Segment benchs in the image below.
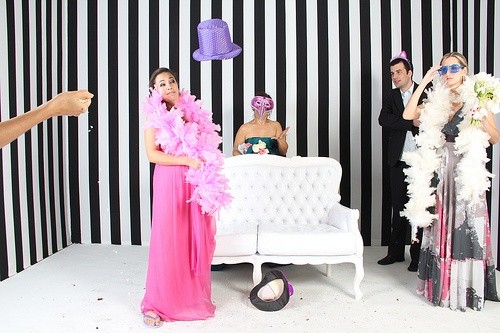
[211,153,364,301]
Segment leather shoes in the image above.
[377,256,406,265]
[407,260,419,271]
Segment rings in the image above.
[85,107,87,110]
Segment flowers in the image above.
[469,78,499,125]
[237,140,269,155]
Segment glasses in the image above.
[438,65,464,75]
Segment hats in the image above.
[249,269,293,312]
[193,19,242,61]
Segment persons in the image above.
[0,90,94,148]
[233,93,290,157]
[140,67,235,329]
[402,53,500,311]
[376,59,427,272]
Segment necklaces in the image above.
[142,86,232,215]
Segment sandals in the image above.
[142,313,160,327]
